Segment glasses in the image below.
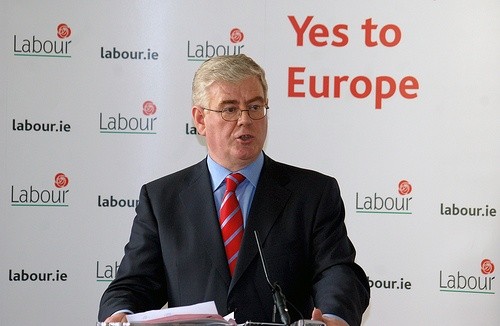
[204,104,269,122]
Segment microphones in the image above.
[254,230,275,290]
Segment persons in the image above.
[98,54,371,326]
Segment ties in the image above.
[219,174,247,276]
